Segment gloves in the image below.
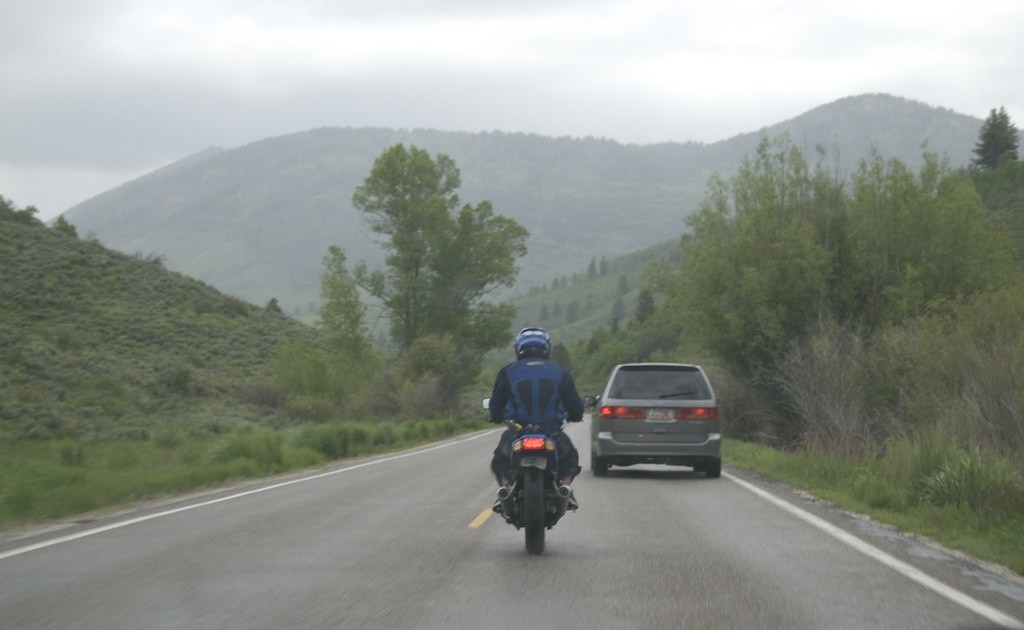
[566,410,581,423]
[489,412,506,424]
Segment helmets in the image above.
[515,326,551,359]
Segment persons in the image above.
[489,327,584,510]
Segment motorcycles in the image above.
[481,394,600,553]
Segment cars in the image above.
[591,363,722,480]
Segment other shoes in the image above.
[492,496,516,511]
[568,492,579,510]
